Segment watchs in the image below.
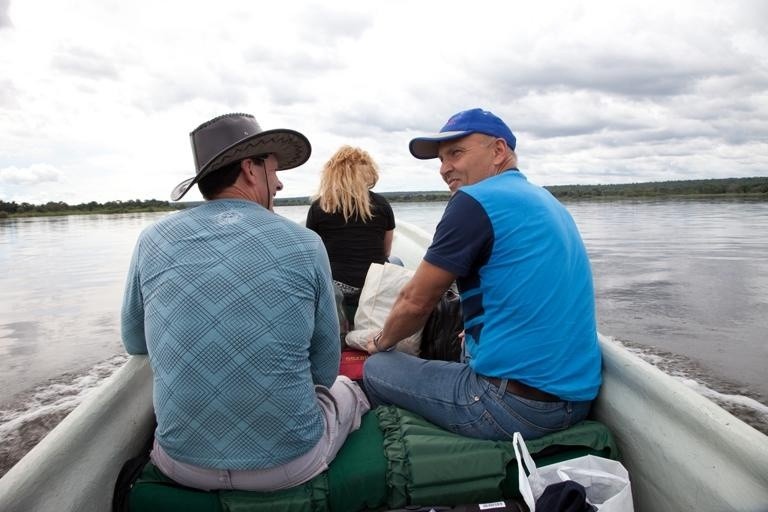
[373,328,396,352]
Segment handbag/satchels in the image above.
[343,262,463,361]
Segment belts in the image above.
[479,374,560,402]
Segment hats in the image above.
[170,113,311,201]
[409,108,516,159]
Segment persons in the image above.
[120,113,371,493]
[363,108,602,441]
[305,144,405,325]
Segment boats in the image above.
[2,217,768,511]
